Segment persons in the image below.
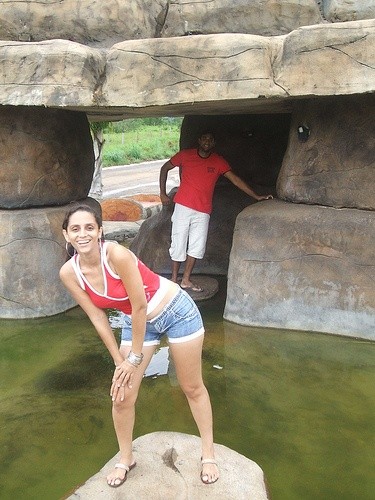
[59,204,219,488]
[159,130,273,292]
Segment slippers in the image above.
[201,457,219,484]
[181,280,204,292]
[176,282,180,285]
[107,459,136,488]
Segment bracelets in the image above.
[128,352,144,366]
